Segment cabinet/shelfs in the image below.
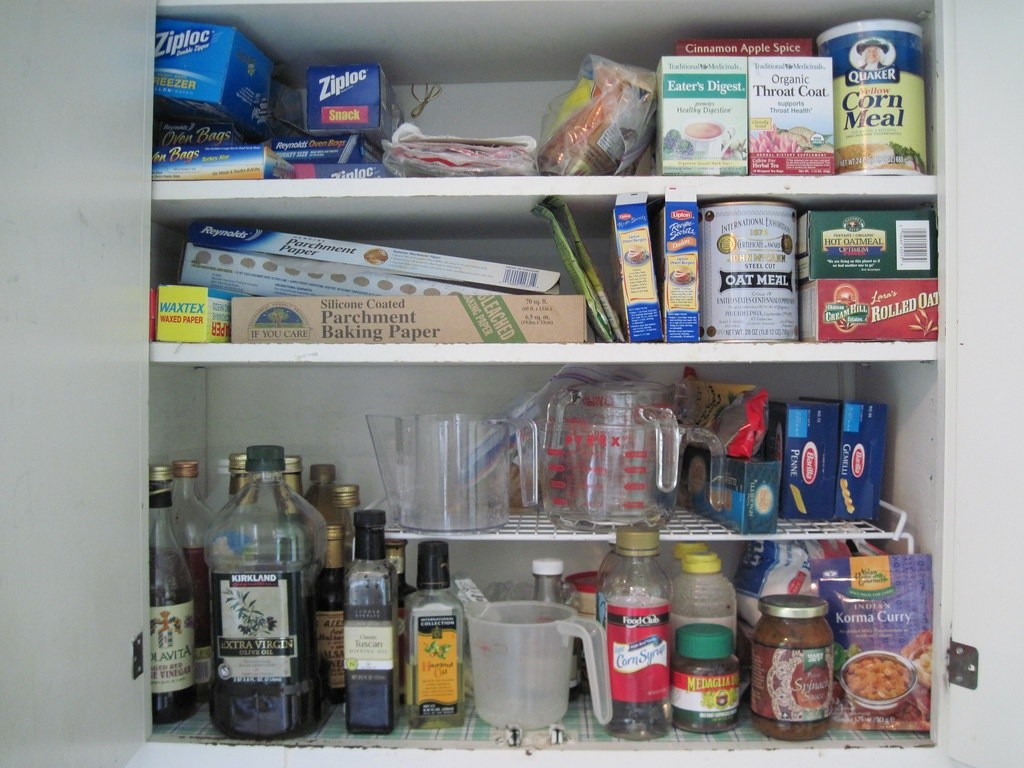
[147,0,942,759]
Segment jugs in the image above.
[545,380,726,527]
[366,412,539,531]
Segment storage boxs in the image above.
[649,16,929,178]
[151,16,405,181]
[677,396,890,536]
[795,208,938,282]
[650,189,702,343]
[615,189,661,341]
[694,199,802,340]
[152,221,598,344]
[798,275,939,342]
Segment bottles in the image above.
[148,444,830,742]
